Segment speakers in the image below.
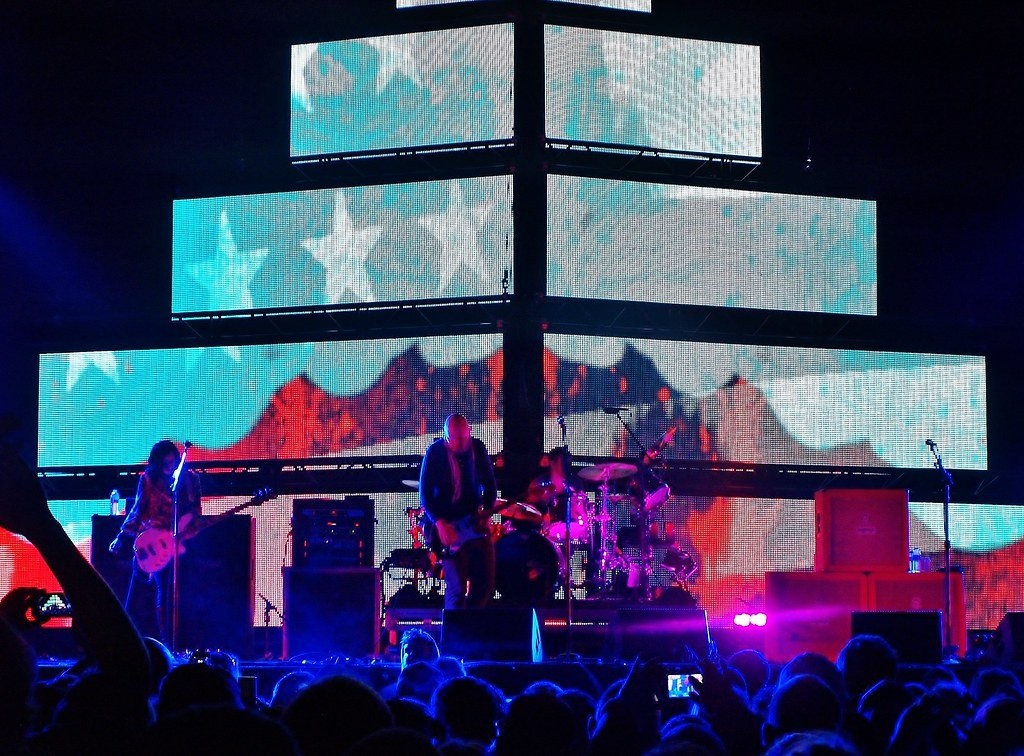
[986,612,1024,667]
[812,489,909,573]
[282,567,383,661]
[851,611,943,665]
[89,515,254,656]
[867,573,966,659]
[764,570,868,662]
[438,608,543,663]
[604,609,711,660]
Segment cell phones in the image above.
[238,677,258,709]
[32,593,73,618]
[663,673,703,699]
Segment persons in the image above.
[0,448,152,756]
[108,440,201,645]
[540,447,590,576]
[419,413,496,609]
[127,589,1024,756]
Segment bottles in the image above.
[909,546,921,572]
[109,489,120,515]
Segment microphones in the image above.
[602,408,628,413]
[926,439,937,445]
[557,415,564,423]
